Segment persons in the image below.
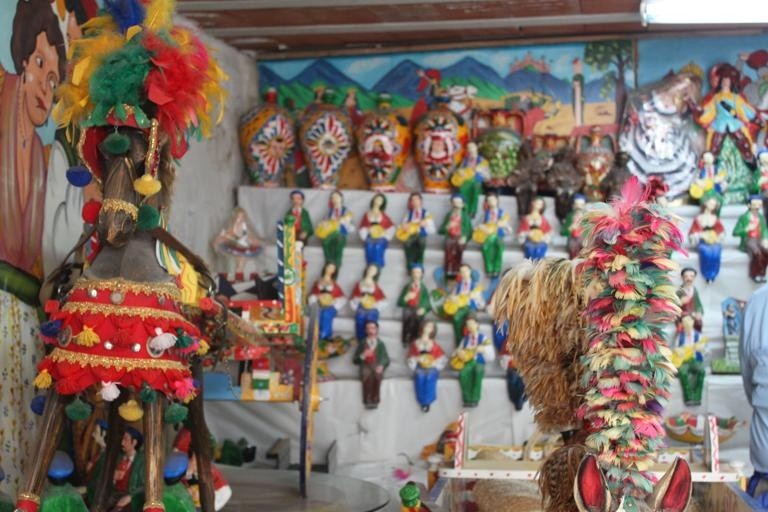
[689,151,727,217]
[675,268,704,333]
[284,191,313,256]
[450,142,490,219]
[443,264,484,348]
[315,189,355,279]
[0,1,68,283]
[397,192,436,273]
[438,193,473,273]
[161,453,201,511]
[397,264,431,348]
[683,61,766,172]
[516,194,553,262]
[353,320,389,410]
[87,421,116,510]
[211,207,266,282]
[472,189,515,279]
[560,193,591,261]
[670,313,708,406]
[688,191,727,285]
[349,264,387,341]
[108,426,147,511]
[359,193,396,268]
[733,195,767,285]
[449,312,496,407]
[406,319,448,414]
[499,332,529,412]
[306,262,349,340]
[738,284,768,472]
[38,450,87,511]
[749,149,768,194]
